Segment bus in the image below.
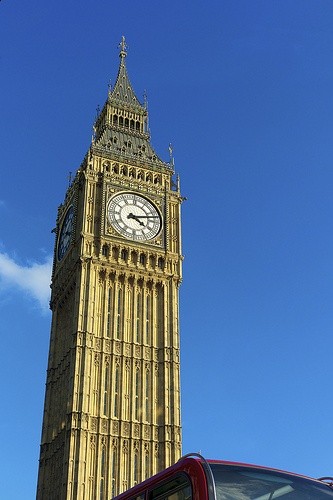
[104,453,331,500]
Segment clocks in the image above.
[107,190,165,242]
[56,204,80,259]
